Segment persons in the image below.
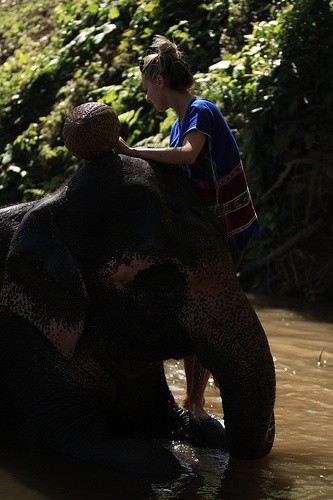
[109,34,264,424]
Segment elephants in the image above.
[0,146,277,484]
